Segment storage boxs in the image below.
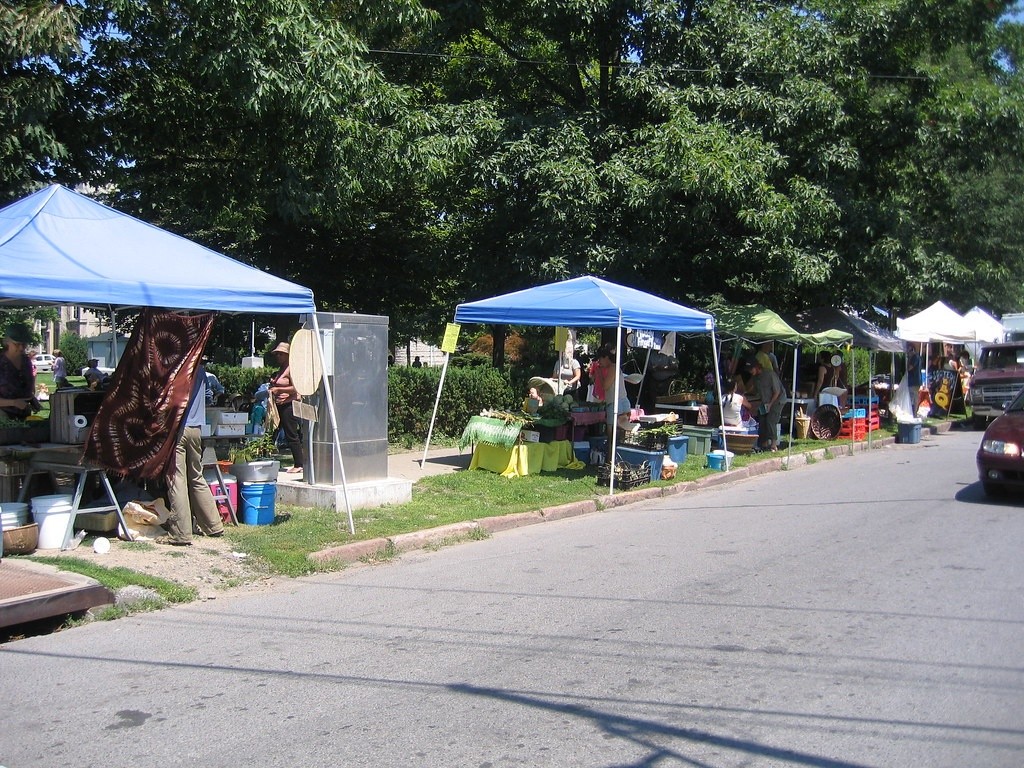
[664,436,689,466]
[835,394,880,441]
[202,468,237,523]
[615,446,667,482]
[201,425,211,436]
[206,411,249,425]
[73,510,119,531]
[211,425,245,436]
[682,425,714,455]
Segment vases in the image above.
[719,433,759,456]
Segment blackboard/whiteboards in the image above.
[930,369,968,417]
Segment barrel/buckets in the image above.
[31,494,75,549]
[240,482,277,525]
[706,450,733,470]
[0,503,39,558]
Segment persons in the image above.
[755,338,779,373]
[48,350,74,389]
[552,355,581,393]
[250,340,304,473]
[814,350,843,408]
[722,380,751,433]
[26,350,38,396]
[904,342,921,417]
[0,323,59,493]
[412,356,422,368]
[84,359,109,389]
[388,355,393,367]
[156,361,226,543]
[522,388,544,406]
[588,342,631,462]
[745,356,787,452]
[917,343,973,419]
[36,382,50,400]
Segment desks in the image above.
[0,428,265,455]
[785,398,817,437]
[654,403,720,426]
[459,410,608,477]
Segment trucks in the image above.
[1000,312,1024,363]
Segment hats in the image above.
[272,342,290,353]
[593,346,610,362]
[7,324,34,343]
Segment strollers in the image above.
[527,376,569,402]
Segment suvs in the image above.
[967,340,1024,430]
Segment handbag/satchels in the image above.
[265,389,280,431]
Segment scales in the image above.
[621,333,645,385]
[821,355,847,398]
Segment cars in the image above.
[33,353,56,366]
[975,386,1024,496]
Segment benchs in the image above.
[15,451,134,543]
[198,440,240,528]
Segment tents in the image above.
[888,301,998,423]
[0,182,355,535]
[964,306,1010,359]
[419,275,729,495]
[798,302,910,452]
[688,290,857,468]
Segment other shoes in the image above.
[288,467,304,473]
[156,534,191,546]
[193,525,224,537]
[769,445,778,453]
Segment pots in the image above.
[229,461,280,481]
